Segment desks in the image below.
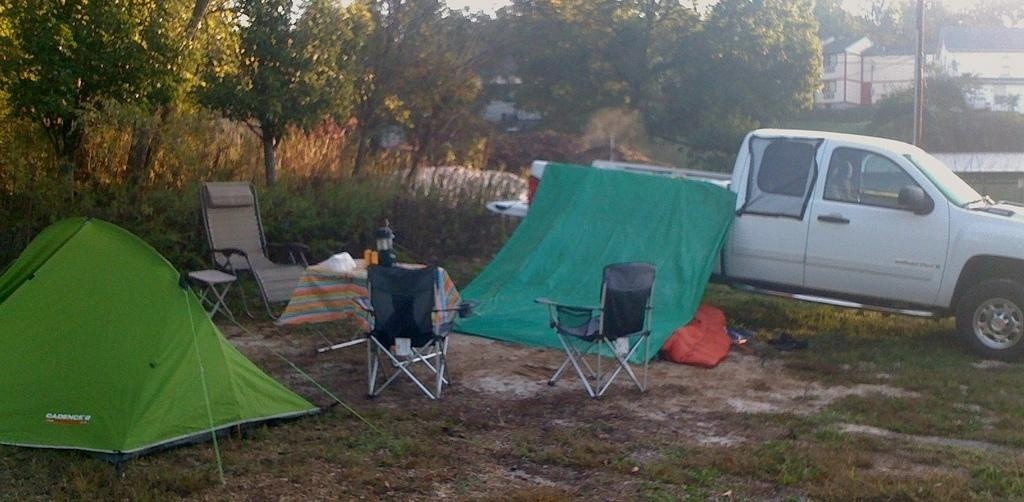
[273,257,465,369]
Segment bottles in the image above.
[363,249,378,270]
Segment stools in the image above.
[186,268,239,325]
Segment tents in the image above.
[1,218,324,458]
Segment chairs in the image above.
[533,260,656,400]
[197,177,315,323]
[351,260,482,404]
[822,161,858,204]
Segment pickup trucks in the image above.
[485,124,1024,364]
